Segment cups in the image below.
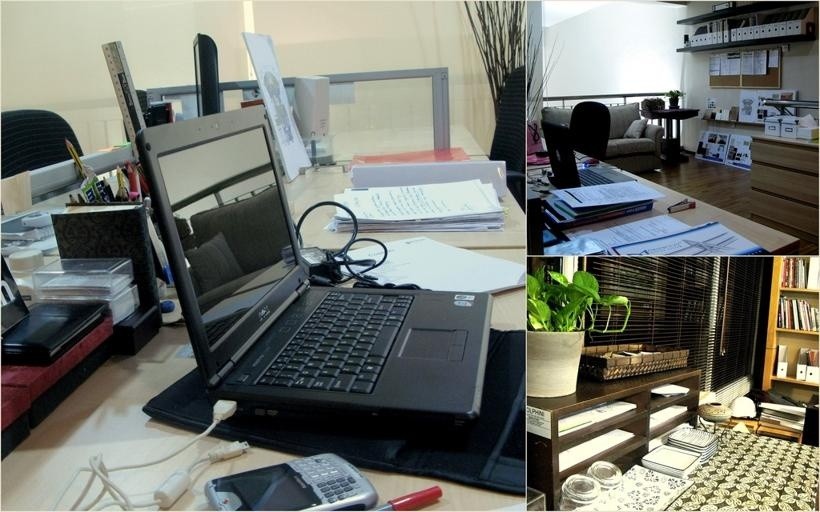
[587,463,624,511]
[561,474,602,512]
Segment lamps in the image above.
[293,74,338,168]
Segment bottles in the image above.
[10,249,47,310]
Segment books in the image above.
[541,183,652,230]
[707,16,754,33]
[778,258,819,366]
[760,401,805,432]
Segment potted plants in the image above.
[664,88,686,109]
[527,259,635,400]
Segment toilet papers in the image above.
[796,114,819,140]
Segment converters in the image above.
[299,246,332,277]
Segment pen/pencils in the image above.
[62,134,148,204]
[376,485,442,511]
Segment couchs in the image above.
[539,99,666,177]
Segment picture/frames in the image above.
[752,88,799,126]
[241,28,312,185]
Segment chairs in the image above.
[489,66,525,214]
[566,101,612,162]
[1,108,87,209]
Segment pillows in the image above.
[623,118,651,140]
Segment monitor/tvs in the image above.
[193,32,221,116]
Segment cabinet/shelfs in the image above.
[746,132,818,239]
[527,362,701,510]
[761,255,819,406]
[673,2,819,57]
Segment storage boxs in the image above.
[764,114,788,137]
[780,115,803,139]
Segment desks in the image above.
[281,151,528,245]
[1,245,527,510]
[528,143,801,254]
[640,108,702,167]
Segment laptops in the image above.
[134,103,494,423]
[541,119,637,190]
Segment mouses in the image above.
[584,158,599,168]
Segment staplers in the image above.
[667,198,696,213]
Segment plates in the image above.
[571,465,692,512]
[641,426,719,478]
